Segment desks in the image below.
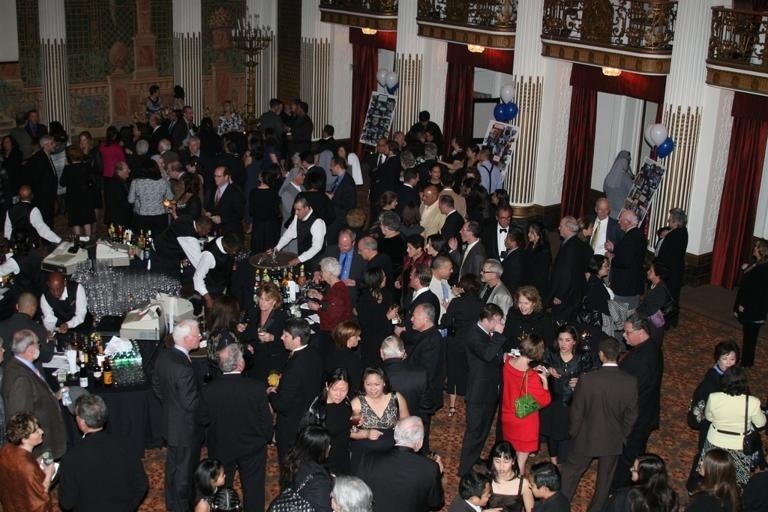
[35,232,184,327]
[38,338,177,464]
[244,250,298,275]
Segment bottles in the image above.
[108,222,151,261]
[42,452,55,465]
[58,382,72,407]
[66,332,112,390]
[251,264,306,303]
[110,336,142,383]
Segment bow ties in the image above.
[499,228,507,234]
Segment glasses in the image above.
[481,268,496,274]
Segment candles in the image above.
[232,8,275,41]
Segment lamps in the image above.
[468,44,485,53]
[601,66,621,79]
[361,27,377,35]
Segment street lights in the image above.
[231,7,277,129]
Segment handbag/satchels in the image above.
[575,277,603,329]
[513,366,543,418]
[266,469,331,512]
[89,140,104,173]
[658,284,681,321]
[743,394,762,456]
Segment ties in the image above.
[442,283,449,299]
[338,251,349,280]
[214,188,220,206]
[590,222,601,250]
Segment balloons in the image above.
[377,68,399,94]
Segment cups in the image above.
[73,258,183,316]
